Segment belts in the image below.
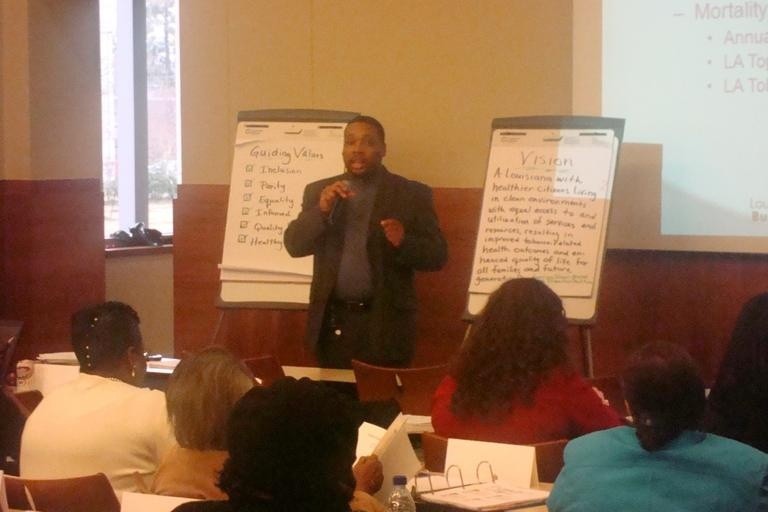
[329,296,370,315]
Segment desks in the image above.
[36,350,357,384]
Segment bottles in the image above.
[384,475,417,512]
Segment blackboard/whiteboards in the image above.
[213,107,361,310]
[459,114,624,327]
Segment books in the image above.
[351,411,435,496]
[403,458,552,512]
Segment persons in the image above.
[163,384,366,512]
[546,336,768,512]
[700,290,768,453]
[152,344,385,512]
[18,300,177,495]
[283,114,449,372]
[429,277,627,444]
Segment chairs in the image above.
[579,375,628,420]
[0,468,123,512]
[421,430,569,484]
[349,357,453,418]
[179,349,287,387]
[13,388,45,413]
